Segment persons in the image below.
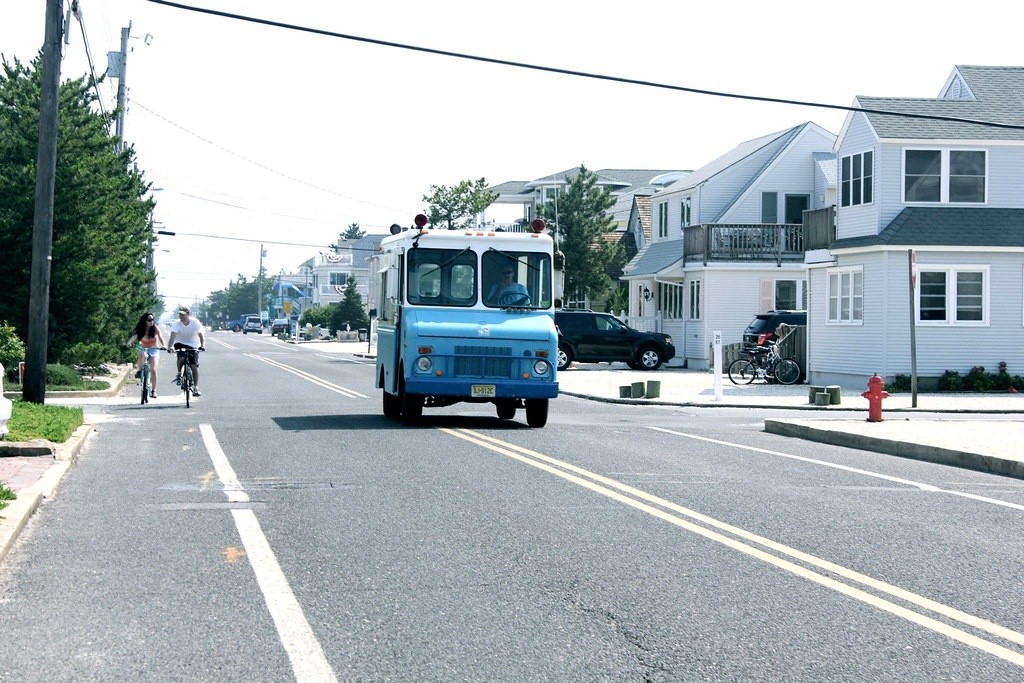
[167,307,205,397]
[487,263,531,306]
[126,313,167,398]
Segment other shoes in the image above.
[193,387,201,396]
[150,390,157,398]
[176,374,182,385]
[135,371,141,378]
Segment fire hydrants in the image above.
[861,371,891,422]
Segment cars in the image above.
[228,315,246,332]
[270,319,291,336]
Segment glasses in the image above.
[502,270,513,275]
[147,319,154,322]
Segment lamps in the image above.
[643,286,654,301]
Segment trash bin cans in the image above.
[358,328,367,342]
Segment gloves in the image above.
[168,347,175,353]
[199,347,205,351]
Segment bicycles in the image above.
[168,347,204,408]
[728,337,801,385]
[130,343,167,405]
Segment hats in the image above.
[177,307,190,314]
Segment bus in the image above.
[375,213,559,428]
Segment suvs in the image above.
[242,315,263,334]
[740,308,807,384]
[554,305,676,371]
